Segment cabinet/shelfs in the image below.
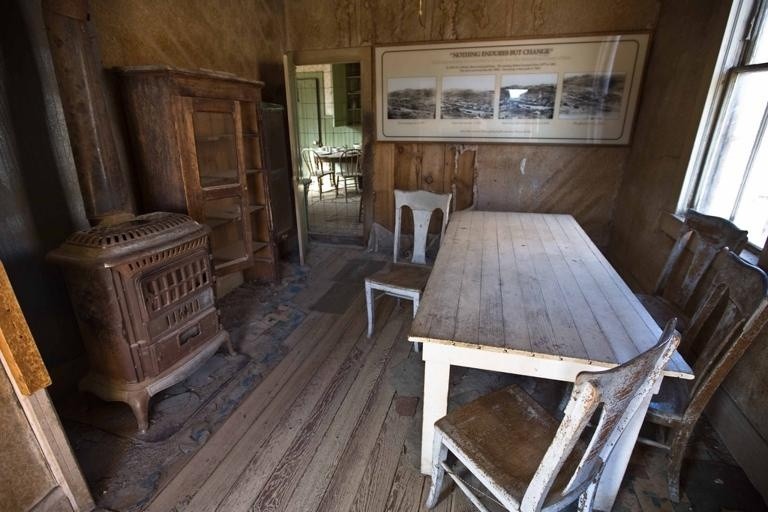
[115,64,279,317]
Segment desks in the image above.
[312,145,363,186]
[409,210,696,510]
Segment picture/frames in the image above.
[373,28,650,149]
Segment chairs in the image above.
[422,313,682,511]
[364,189,452,353]
[632,212,768,503]
[301,146,363,203]
